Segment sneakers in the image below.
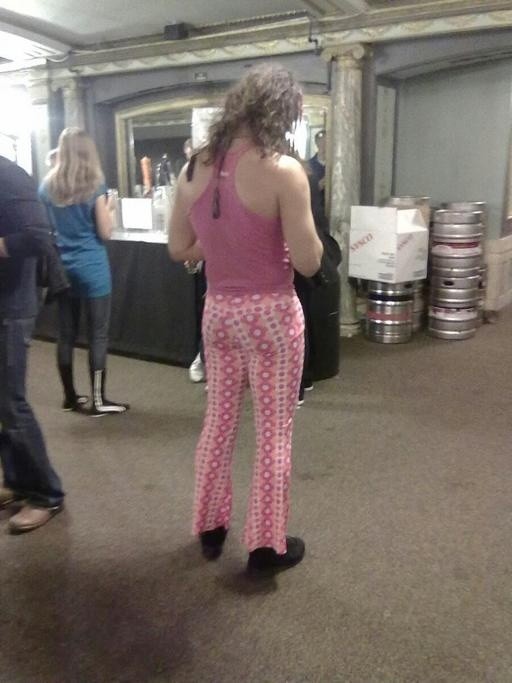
[9,501,65,531]
[189,353,206,383]
[297,373,314,405]
[0,486,30,508]
[247,535,304,581]
[198,524,229,560]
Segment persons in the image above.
[168,62,326,580]
[0,155,71,532]
[39,126,128,420]
[296,144,317,406]
[308,132,326,202]
[189,339,208,385]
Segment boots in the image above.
[88,368,130,418]
[61,369,88,411]
